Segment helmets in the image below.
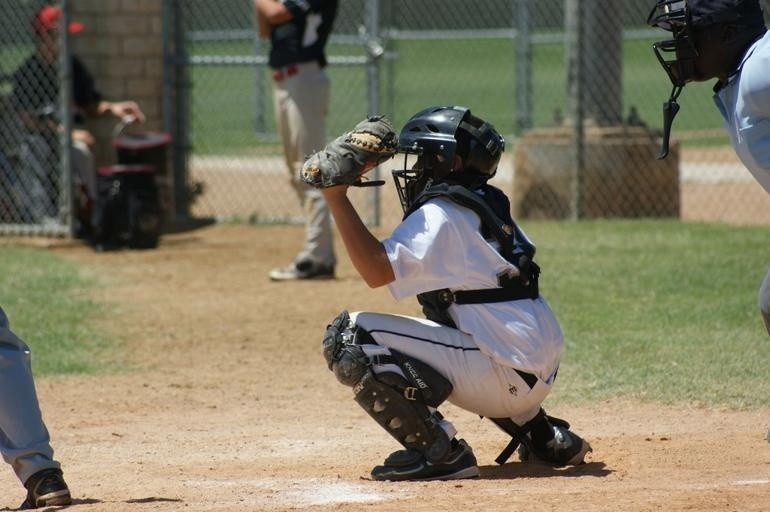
[392,106,505,214]
[647,0,768,87]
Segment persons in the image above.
[251,0,342,283]
[643,0,769,337]
[0,305,73,511]
[298,104,593,481]
[10,5,147,240]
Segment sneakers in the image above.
[18,468,72,510]
[268,253,335,280]
[519,419,593,466]
[369,437,479,483]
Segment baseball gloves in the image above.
[300,114,398,187]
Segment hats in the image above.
[32,6,84,36]
